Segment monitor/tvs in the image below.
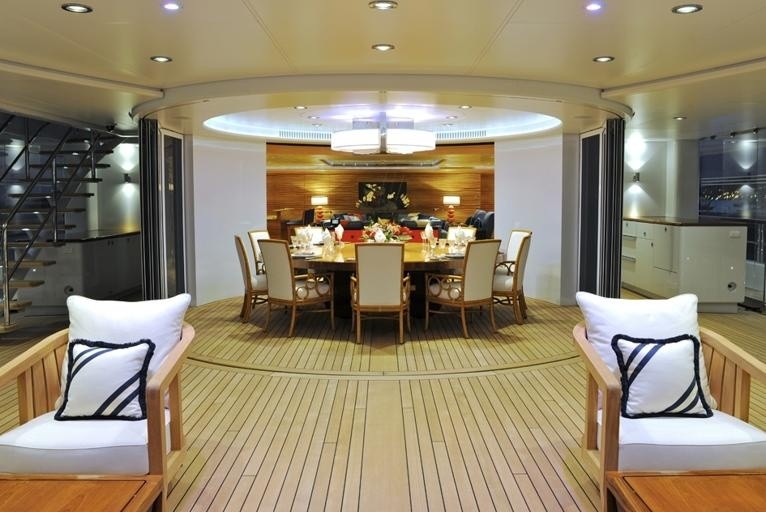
[359,182,407,210]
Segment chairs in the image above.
[0,321,195,512]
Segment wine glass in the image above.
[421,226,476,263]
[289,227,344,258]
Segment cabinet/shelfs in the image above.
[572,319,766,512]
[621,221,747,304]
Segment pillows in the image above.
[55,293,191,413]
[54,339,156,421]
[612,333,713,419]
[574,291,718,411]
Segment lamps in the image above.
[633,172,639,182]
[330,112,436,155]
